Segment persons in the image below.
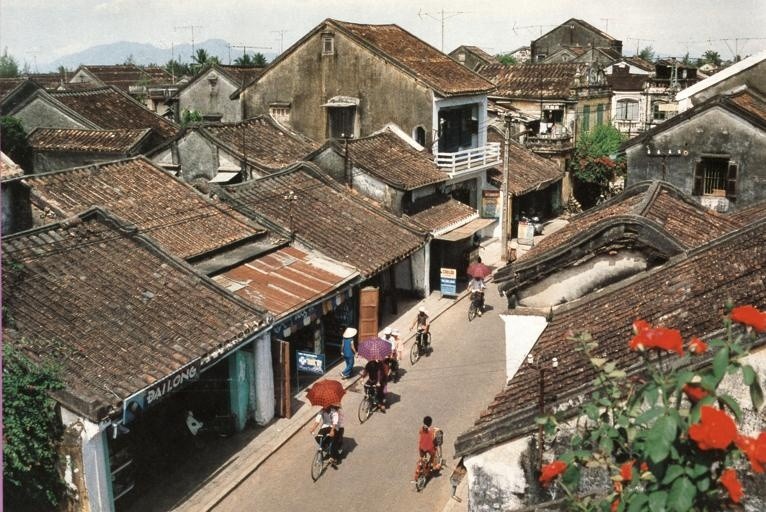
[409,307,430,357]
[466,277,483,317]
[413,415,440,482]
[328,406,344,463]
[340,327,357,378]
[381,327,397,376]
[391,328,403,368]
[359,361,382,408]
[308,406,338,465]
[375,360,388,412]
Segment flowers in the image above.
[536,297,766,511]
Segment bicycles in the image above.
[412,427,443,490]
[408,326,432,366]
[358,375,388,423]
[465,288,487,321]
[309,429,344,482]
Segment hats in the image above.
[384,327,399,336]
[342,327,357,339]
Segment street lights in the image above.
[283,188,299,247]
[500,126,533,262]
[338,132,354,188]
[527,352,558,469]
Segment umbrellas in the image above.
[466,261,491,278]
[304,379,346,410]
[357,337,392,362]
